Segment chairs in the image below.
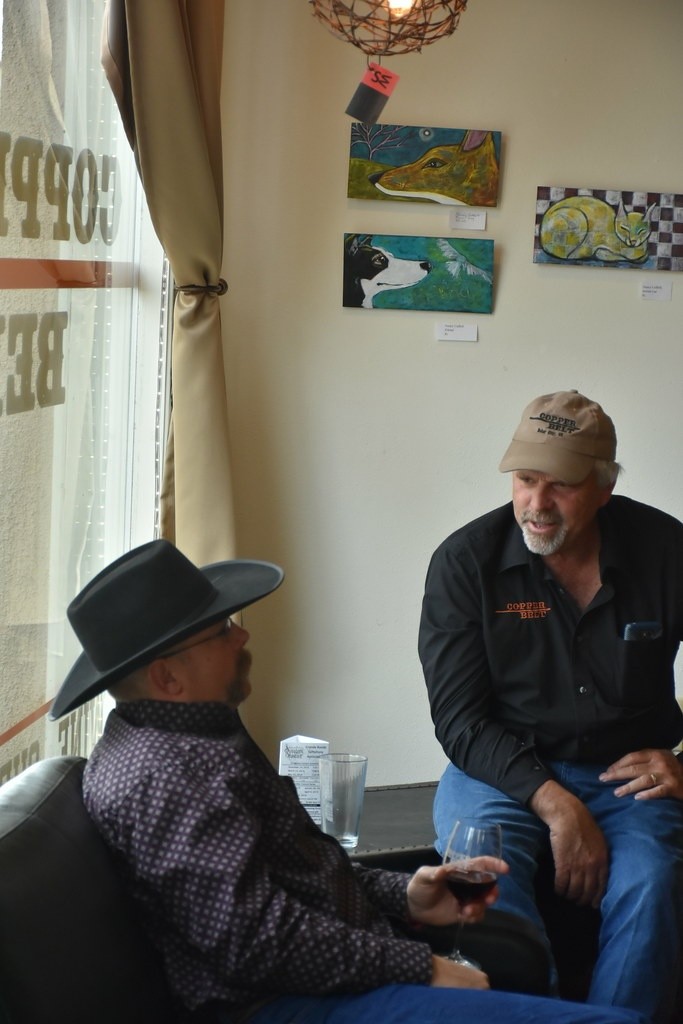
[0,754,207,1024]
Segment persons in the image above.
[414,387,683,1010]
[44,539,653,1024]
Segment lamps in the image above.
[310,0,468,57]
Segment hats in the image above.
[48,539,284,721]
[499,390,618,484]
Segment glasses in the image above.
[147,618,231,664]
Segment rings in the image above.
[651,773,657,786]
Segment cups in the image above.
[319,753,368,848]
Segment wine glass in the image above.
[443,818,503,974]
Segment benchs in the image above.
[319,781,444,874]
